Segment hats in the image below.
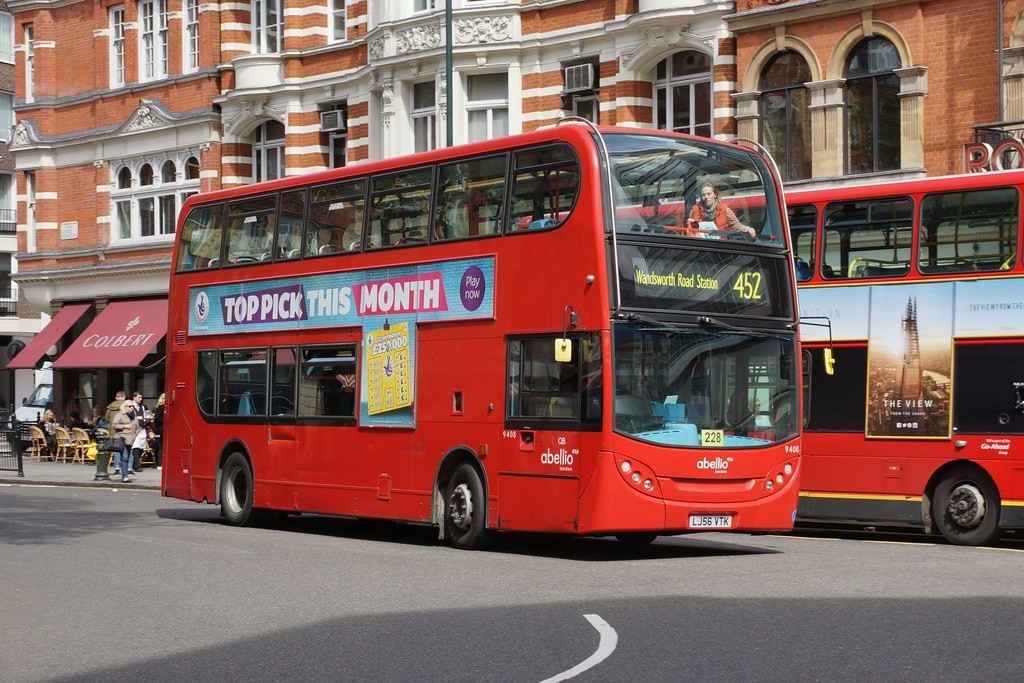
[69,411,79,418]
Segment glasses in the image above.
[126,406,134,409]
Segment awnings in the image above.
[5,297,168,367]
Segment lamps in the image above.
[45,344,57,362]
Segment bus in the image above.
[159,114,836,550]
[516,168,1023,547]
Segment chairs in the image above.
[29,426,56,463]
[96,428,155,469]
[207,239,375,267]
[54,428,97,465]
[794,254,1016,282]
[237,388,296,416]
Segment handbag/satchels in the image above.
[105,437,125,451]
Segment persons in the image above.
[686,181,755,242]
[36,391,165,483]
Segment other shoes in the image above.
[114,470,119,475]
[135,468,143,472]
[157,465,162,470]
[122,477,130,483]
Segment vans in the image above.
[7,383,53,452]
